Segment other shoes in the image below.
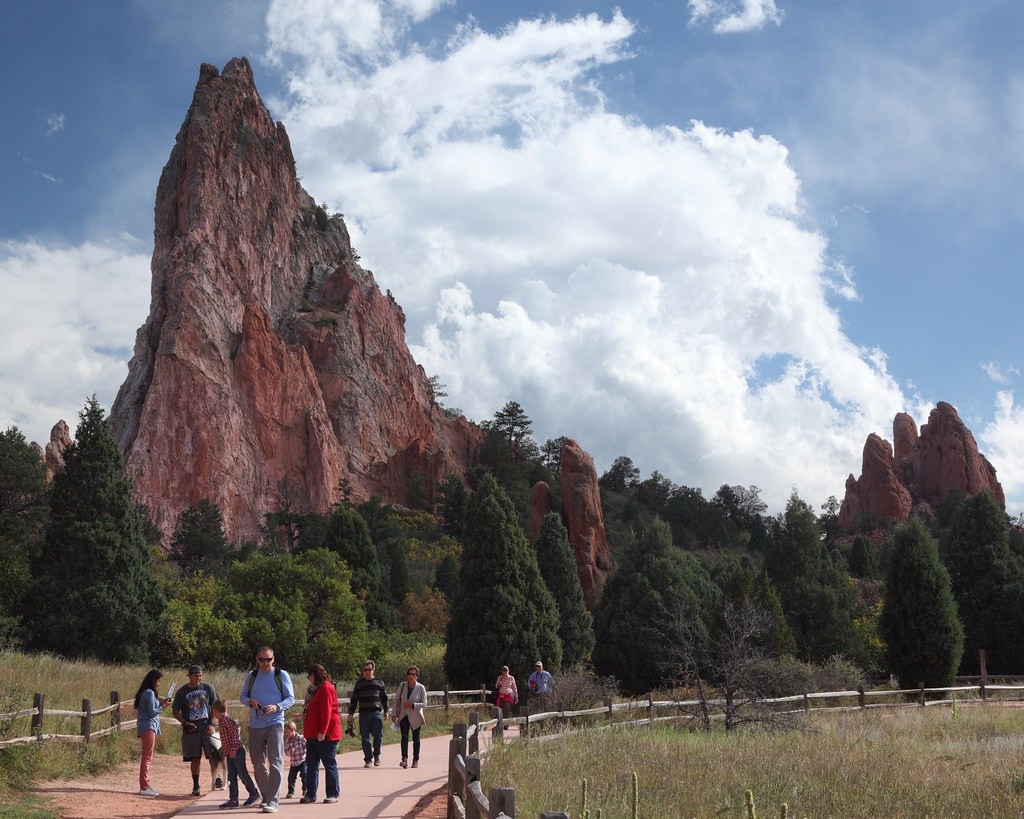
[260,798,267,808]
[364,762,371,768]
[215,778,223,788]
[324,797,338,803]
[412,759,418,768]
[400,762,407,769]
[286,793,293,798]
[263,802,278,812]
[243,792,260,808]
[139,787,159,796]
[219,800,240,809]
[192,786,200,794]
[300,796,315,803]
[375,755,380,766]
[303,789,307,795]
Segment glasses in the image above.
[307,673,313,680]
[258,656,273,663]
[503,670,508,672]
[408,672,417,676]
[363,668,373,673]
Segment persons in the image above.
[346,660,389,767]
[134,646,342,813]
[526,662,556,697]
[497,667,519,731]
[390,665,428,769]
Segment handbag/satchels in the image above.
[491,675,502,700]
[396,682,405,718]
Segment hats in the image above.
[186,665,202,676]
[535,661,542,666]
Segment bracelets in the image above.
[277,705,280,712]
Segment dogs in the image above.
[208,731,230,791]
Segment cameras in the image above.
[345,723,355,737]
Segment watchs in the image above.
[182,720,187,726]
